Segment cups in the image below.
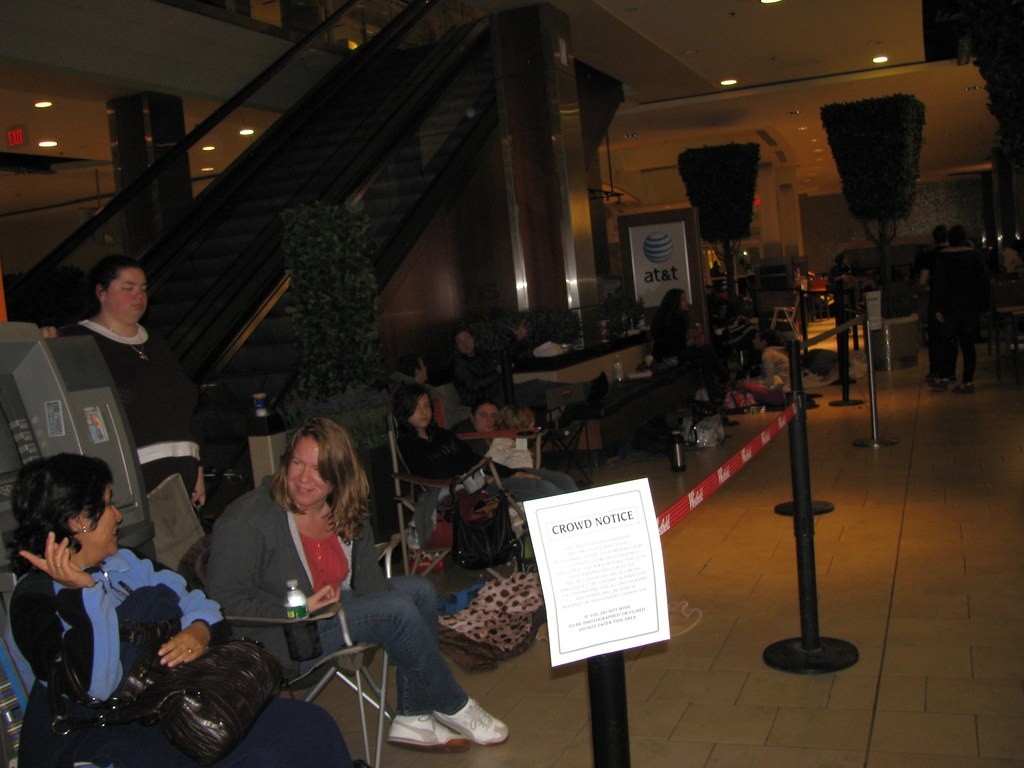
[597,320,610,343]
[252,389,267,416]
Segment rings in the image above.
[55,564,60,567]
[187,648,193,653]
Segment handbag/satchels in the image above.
[48,619,282,768]
[451,478,520,570]
[679,412,726,449]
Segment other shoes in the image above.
[928,380,948,391]
[953,383,974,393]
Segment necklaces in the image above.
[97,315,144,359]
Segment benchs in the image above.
[568,361,699,460]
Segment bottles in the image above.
[284,580,309,619]
[613,352,624,382]
[694,322,704,347]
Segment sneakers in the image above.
[433,695,508,747]
[385,710,472,752]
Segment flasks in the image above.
[671,430,686,472]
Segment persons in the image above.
[914,224,991,394]
[650,275,792,414]
[6,453,356,767]
[1002,233,1024,280]
[392,384,579,505]
[829,251,863,289]
[204,417,509,754]
[415,319,608,414]
[39,254,206,513]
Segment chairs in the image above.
[769,292,807,346]
[381,403,547,586]
[515,377,591,484]
[145,472,400,768]
[987,275,1024,385]
